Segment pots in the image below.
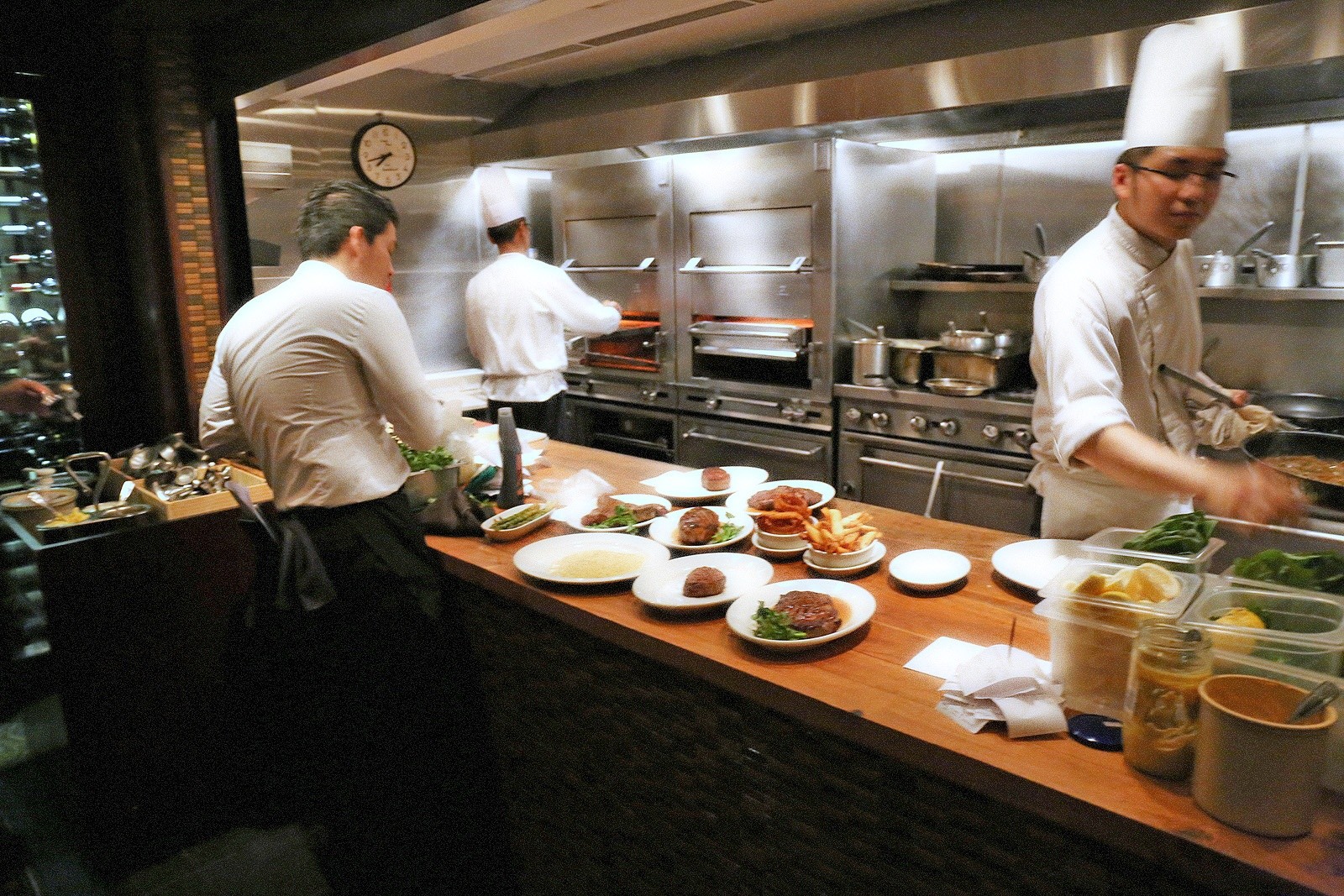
[1313,241,1344,287]
[1192,249,1247,289]
[851,339,893,387]
[888,348,933,385]
[1245,393,1344,433]
[1183,397,1344,514]
[1021,248,1062,282]
[979,310,1022,348]
[941,321,995,352]
[1250,249,1317,289]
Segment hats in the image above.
[480,169,528,229]
[1115,25,1231,164]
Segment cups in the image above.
[1192,674,1341,836]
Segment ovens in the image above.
[549,136,937,403]
[550,374,836,497]
[836,429,1047,538]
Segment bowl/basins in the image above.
[750,530,809,560]
[809,539,877,568]
[802,540,888,580]
[755,516,820,550]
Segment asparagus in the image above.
[491,502,556,532]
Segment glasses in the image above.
[1126,164,1239,191]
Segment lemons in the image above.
[1068,561,1179,614]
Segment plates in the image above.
[990,538,1093,592]
[889,549,972,592]
[726,578,877,653]
[647,505,754,553]
[655,466,770,505]
[481,502,556,542]
[924,377,990,396]
[632,552,774,615]
[726,480,835,515]
[512,532,672,585]
[566,493,672,533]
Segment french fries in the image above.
[801,506,882,554]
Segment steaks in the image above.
[677,507,719,544]
[772,589,843,638]
[582,491,667,524]
[700,467,733,491]
[748,485,822,512]
[684,564,728,597]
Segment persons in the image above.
[1024,22,1313,552]
[466,163,625,440]
[192,177,454,896]
[0,374,59,416]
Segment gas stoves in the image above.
[834,371,1046,460]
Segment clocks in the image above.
[351,121,417,191]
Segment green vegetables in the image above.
[396,442,456,471]
[712,520,743,544]
[1102,509,1343,600]
[584,505,638,535]
[752,602,806,641]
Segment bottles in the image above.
[496,406,524,508]
[1120,616,1214,782]
